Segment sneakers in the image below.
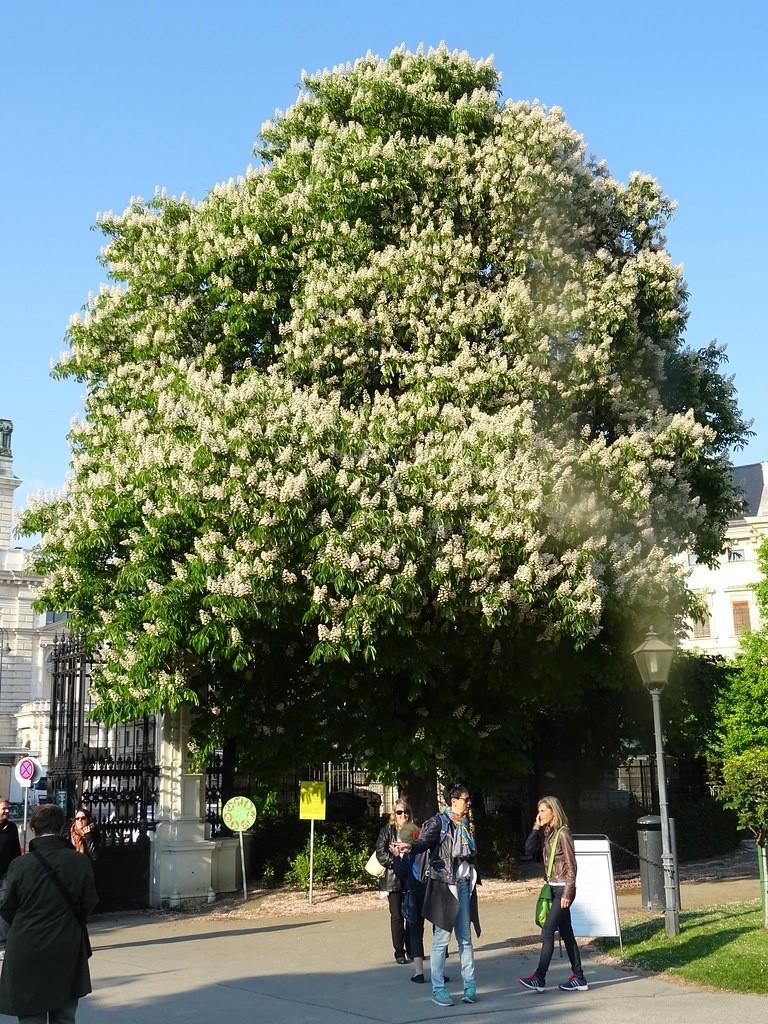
[461,987,476,1003]
[559,975,588,991]
[431,988,455,1006]
[519,973,546,992]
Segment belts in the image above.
[457,877,470,882]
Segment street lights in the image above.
[630,625,680,938]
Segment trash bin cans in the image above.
[636,815,667,911]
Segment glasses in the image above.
[74,816,87,821]
[395,808,410,815]
[451,797,473,803]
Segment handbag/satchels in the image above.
[411,812,455,886]
[400,888,417,923]
[365,850,386,878]
[535,882,553,928]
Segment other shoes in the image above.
[444,976,449,983]
[396,957,407,964]
[410,974,424,983]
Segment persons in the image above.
[70,808,98,868]
[376,782,477,1006]
[516,795,590,993]
[0,803,98,1024]
[0,798,22,961]
